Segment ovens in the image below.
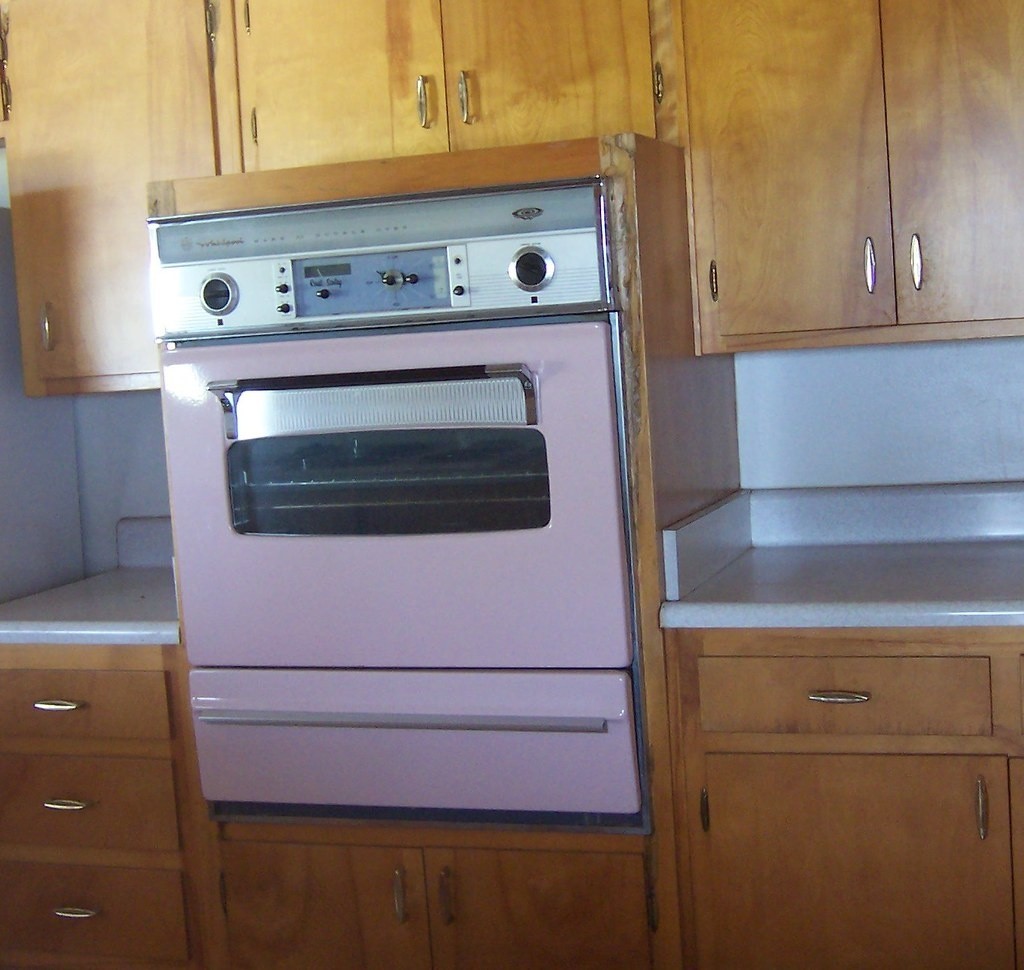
[153,180,644,817]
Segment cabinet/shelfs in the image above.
[0,621,1023,970]
[1,1,1024,400]
